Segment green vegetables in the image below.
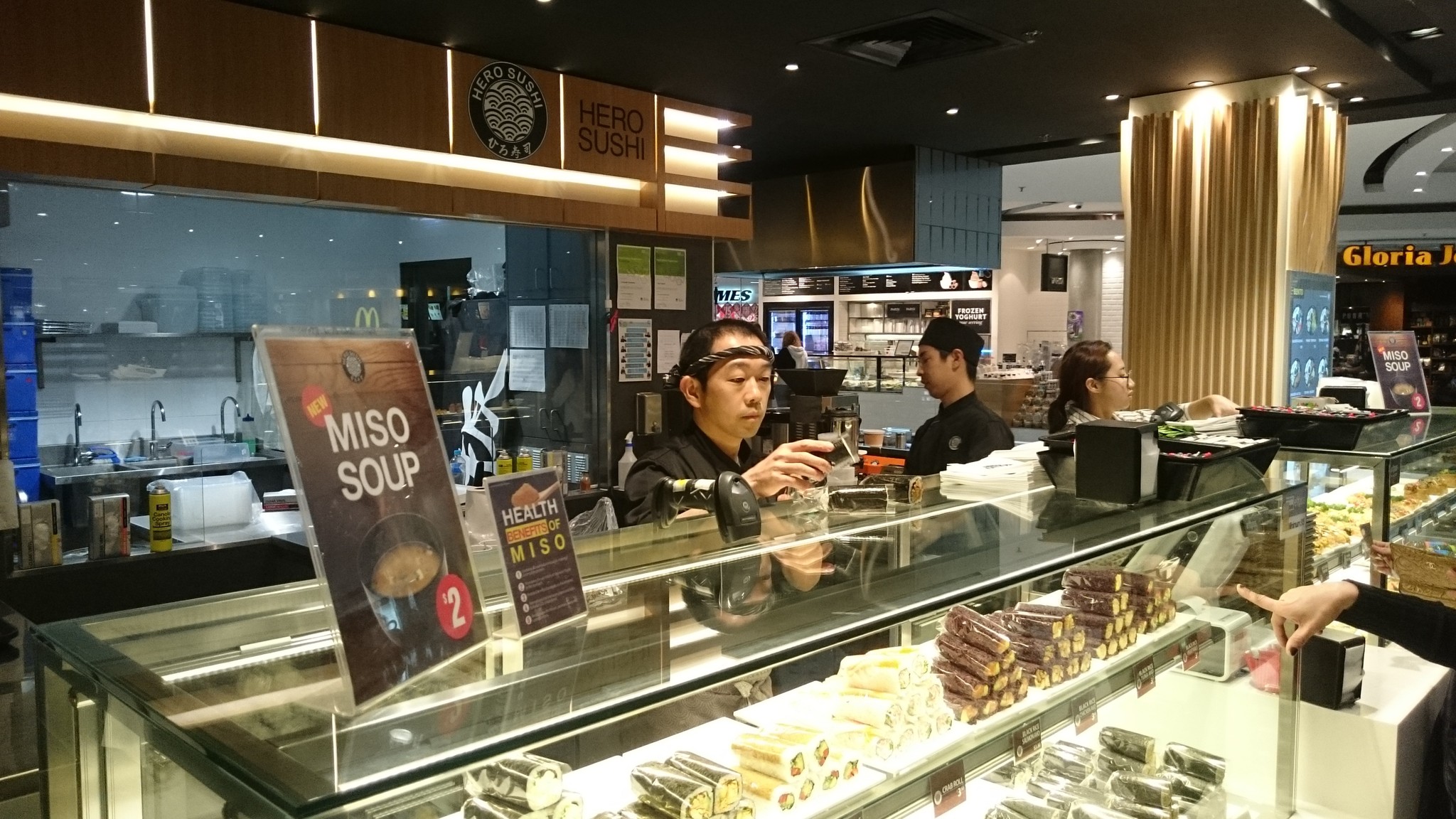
[1306,493,1405,521]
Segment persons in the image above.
[773,331,809,369]
[911,500,999,558]
[625,318,834,526]
[902,316,1014,478]
[1368,539,1456,610]
[680,507,836,634]
[1048,340,1239,437]
[1237,577,1456,671]
[1131,554,1238,636]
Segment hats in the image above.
[918,316,985,368]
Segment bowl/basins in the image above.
[1390,376,1418,409]
[357,512,448,648]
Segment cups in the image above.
[969,279,978,289]
[863,429,885,447]
[940,280,951,289]
[263,430,275,449]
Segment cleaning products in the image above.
[617,431,637,490]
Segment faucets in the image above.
[74,402,100,467]
[149,400,173,460]
[220,395,241,443]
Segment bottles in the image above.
[450,450,466,485]
[517,448,533,471]
[149,480,173,552]
[896,432,907,448]
[496,449,513,476]
[243,413,257,455]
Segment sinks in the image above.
[39,462,141,485]
[191,450,285,464]
[116,457,200,471]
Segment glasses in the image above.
[1087,370,1133,388]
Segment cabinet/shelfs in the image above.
[763,271,996,395]
[0,406,1456,819]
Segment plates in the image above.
[1311,476,1456,524]
[431,586,1198,818]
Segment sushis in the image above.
[461,568,1227,819]
[827,484,889,513]
[801,437,855,481]
[863,473,923,504]
